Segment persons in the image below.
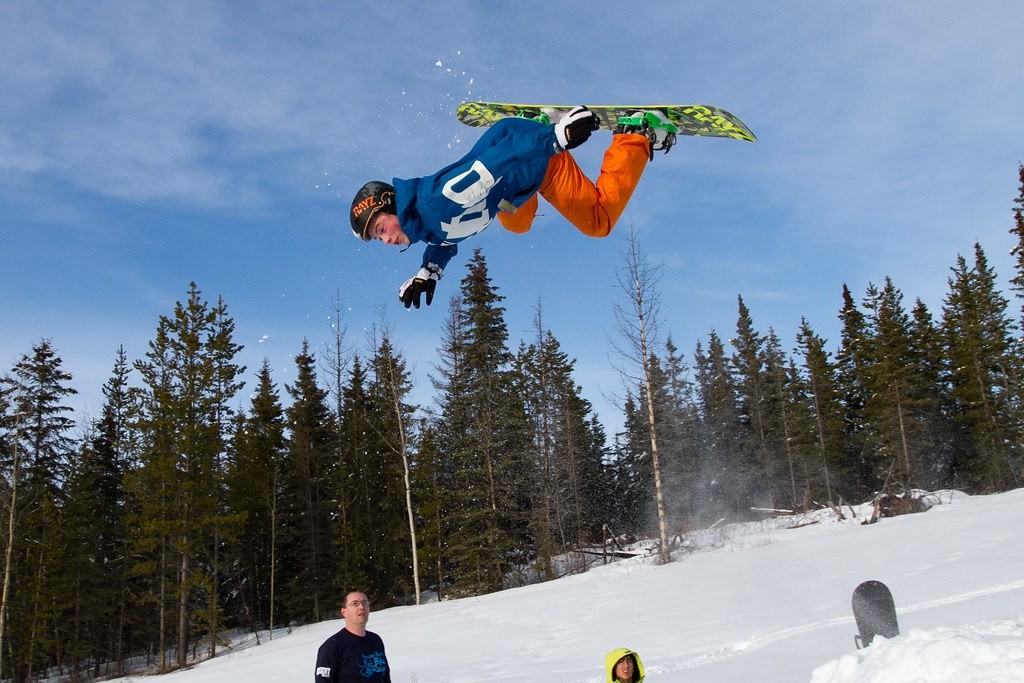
[315,587,392,683]
[350,105,678,309]
[605,648,646,683]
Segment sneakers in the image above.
[516,108,549,126]
[612,109,679,162]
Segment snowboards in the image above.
[456,101,759,144]
[851,580,900,648]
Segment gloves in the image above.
[554,105,600,151]
[399,268,439,308]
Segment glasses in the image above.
[345,600,371,607]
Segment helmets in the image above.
[350,181,396,241]
